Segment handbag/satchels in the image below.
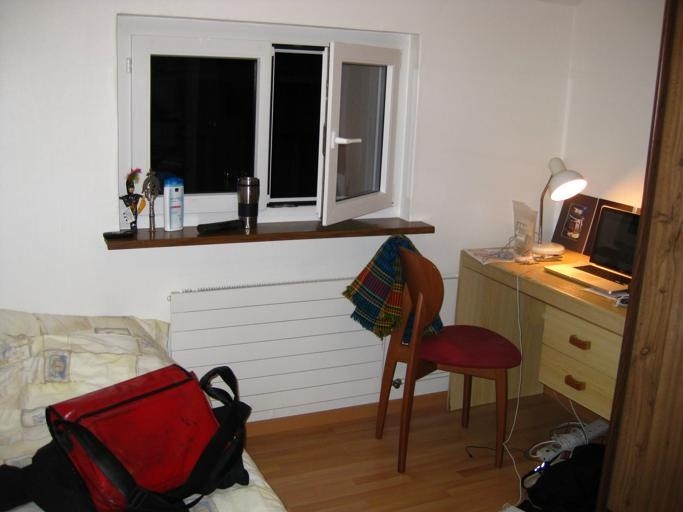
[523,441,604,508]
[1,361,252,512]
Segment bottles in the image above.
[236,176,260,233]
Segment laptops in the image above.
[543,205,641,296]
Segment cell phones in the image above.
[103,231,134,239]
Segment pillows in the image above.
[0,310,170,462]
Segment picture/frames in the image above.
[550,193,598,254]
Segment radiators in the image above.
[166,277,459,424]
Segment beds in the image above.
[0,446,287,512]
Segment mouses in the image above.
[616,296,630,307]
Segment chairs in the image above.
[375,245,521,474]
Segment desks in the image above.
[444,242,629,422]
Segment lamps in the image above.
[531,157,587,255]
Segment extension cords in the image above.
[536,419,609,462]
